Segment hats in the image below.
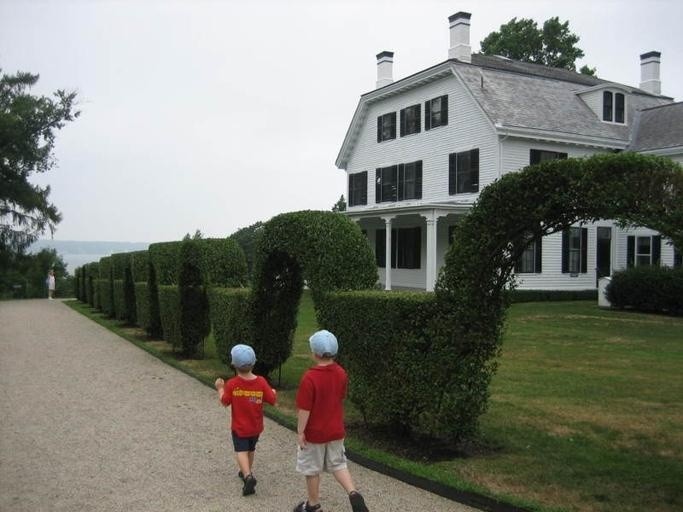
[309,329,339,359]
[230,344,257,369]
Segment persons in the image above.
[215,344,277,496]
[48,271,56,300]
[294,329,369,512]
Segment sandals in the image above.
[238,471,258,496]
[293,500,323,512]
[349,491,369,512]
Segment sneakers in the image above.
[47,296,55,301]
